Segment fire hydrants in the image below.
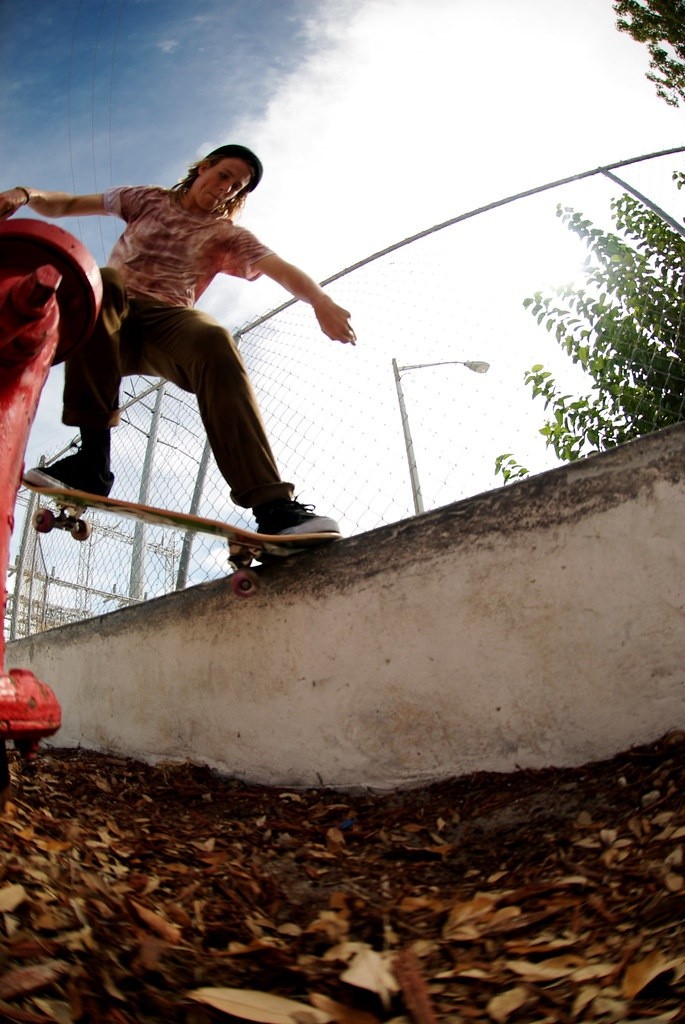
[1,215,107,735]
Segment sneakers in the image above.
[22,443,114,499]
[254,498,338,534]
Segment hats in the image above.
[190,145,261,192]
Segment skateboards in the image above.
[19,467,340,596]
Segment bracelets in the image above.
[15,186,30,205]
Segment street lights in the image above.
[389,358,492,512]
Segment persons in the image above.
[0,143,360,539]
